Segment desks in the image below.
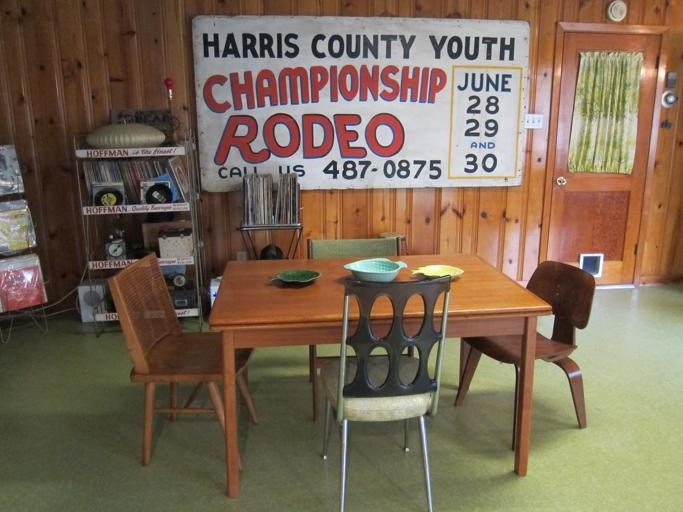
[209,254,552,498]
[237,227,303,259]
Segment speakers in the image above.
[78,277,108,323]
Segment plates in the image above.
[410,264,464,281]
[271,269,321,284]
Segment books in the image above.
[83,159,163,204]
[244,173,299,229]
[0,143,48,312]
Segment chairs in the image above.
[108,252,260,471]
[307,237,413,421]
[455,261,595,451]
[321,275,451,512]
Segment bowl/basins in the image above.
[343,258,408,283]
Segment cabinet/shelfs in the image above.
[74,131,208,338]
